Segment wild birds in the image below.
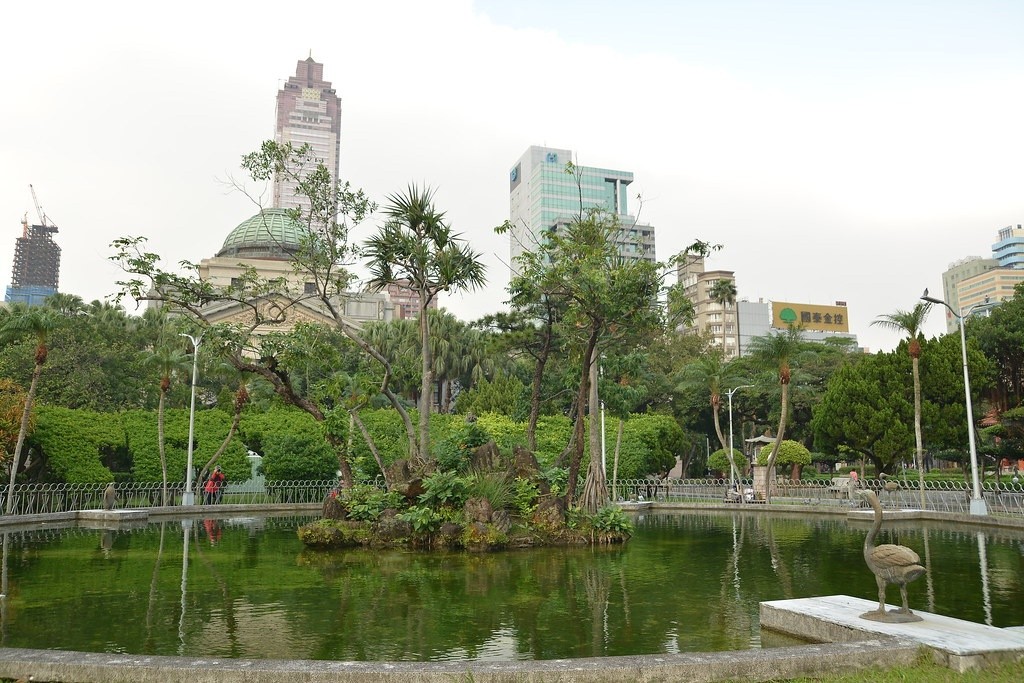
[854,488,928,617]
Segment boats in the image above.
[616,500,653,511]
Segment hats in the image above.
[215,466,221,470]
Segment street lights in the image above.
[177,329,209,505]
[724,385,756,499]
[920,295,996,517]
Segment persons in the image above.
[724,479,755,500]
[203,465,227,504]
[850,468,857,481]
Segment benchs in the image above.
[827,477,851,500]
